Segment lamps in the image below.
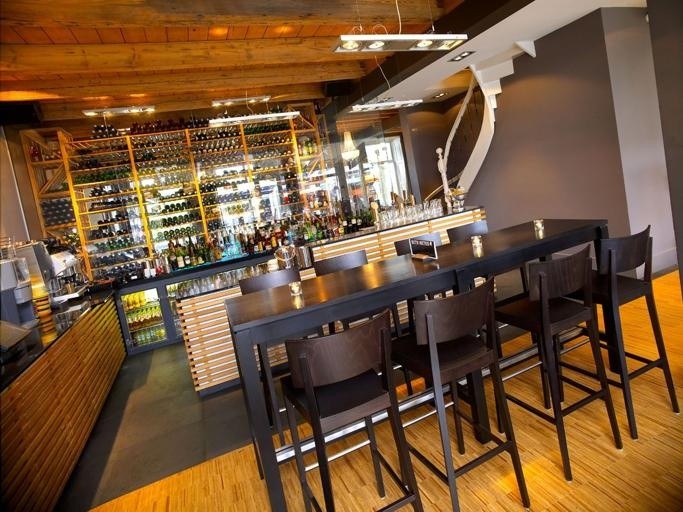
[330,1,469,53]
[341,131,361,162]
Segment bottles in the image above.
[375,198,443,228]
[24,101,378,288]
[120,263,260,347]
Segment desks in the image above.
[224,218,632,512]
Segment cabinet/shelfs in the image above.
[126,129,210,258]
[283,102,331,217]
[241,118,309,218]
[21,127,88,282]
[186,121,260,243]
[57,132,156,282]
[114,253,277,355]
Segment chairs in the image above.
[567,226,681,438]
[390,276,531,512]
[490,243,623,481]
[395,231,441,256]
[279,309,424,512]
[447,219,488,243]
[239,268,299,294]
[314,250,368,276]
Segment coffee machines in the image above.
[49,250,91,300]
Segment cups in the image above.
[1,233,16,259]
